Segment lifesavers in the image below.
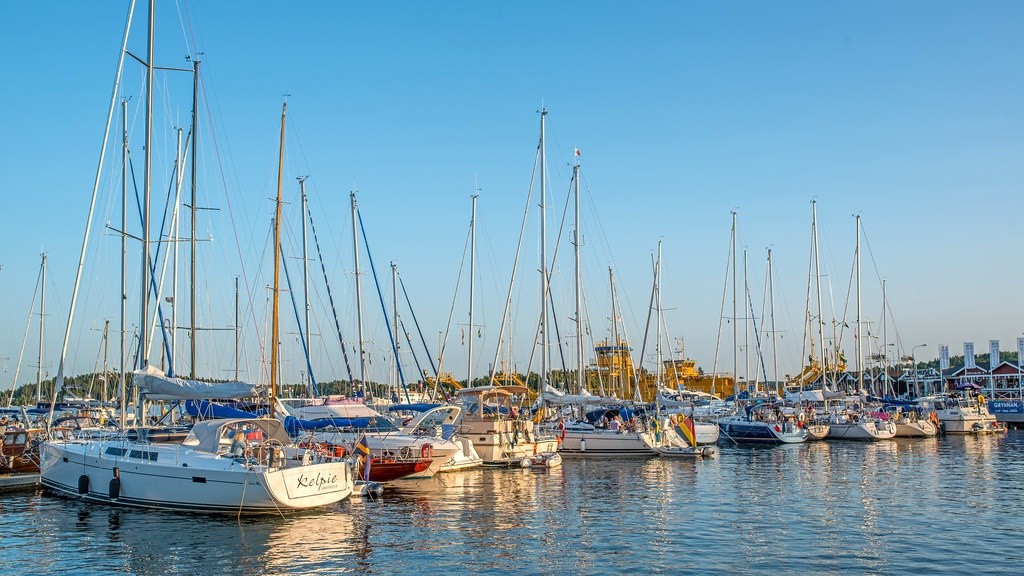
[513,405,518,416]
[978,395,985,402]
[799,413,805,421]
[676,414,685,423]
[420,444,434,459]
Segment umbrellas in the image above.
[955,382,981,398]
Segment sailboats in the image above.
[0,1,1002,516]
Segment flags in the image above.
[559,418,565,441]
[351,432,370,485]
[677,411,697,450]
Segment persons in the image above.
[1015,380,1018,388]
[392,391,398,403]
[997,381,1003,388]
[0,417,9,456]
[805,399,817,425]
[230,426,247,453]
[973,388,978,397]
